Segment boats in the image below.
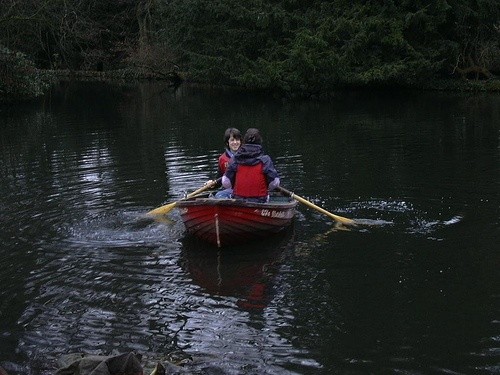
[176,192,299,247]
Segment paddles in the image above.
[148,175,222,214]
[275,184,353,226]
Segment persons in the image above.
[202,127,281,203]
